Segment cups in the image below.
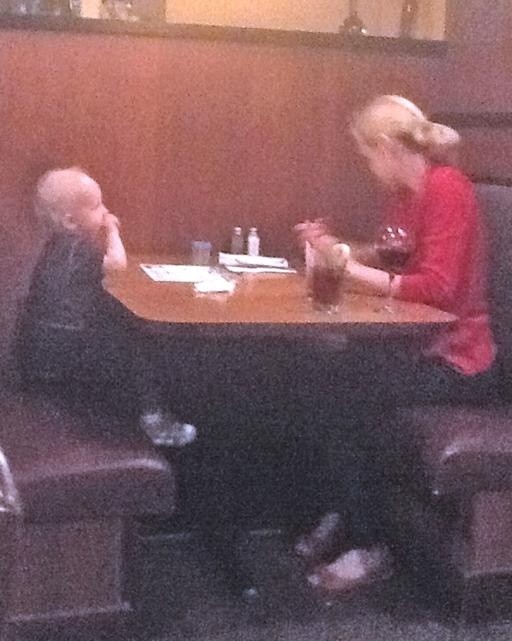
[190,240,212,264]
[306,236,349,312]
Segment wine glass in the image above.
[373,225,413,314]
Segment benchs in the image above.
[377,180,512,628]
[0,379,177,629]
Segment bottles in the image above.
[230,224,260,256]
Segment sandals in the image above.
[305,542,396,594]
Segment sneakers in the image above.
[138,411,198,447]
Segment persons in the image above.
[293,92,499,595]
[7,166,198,448]
